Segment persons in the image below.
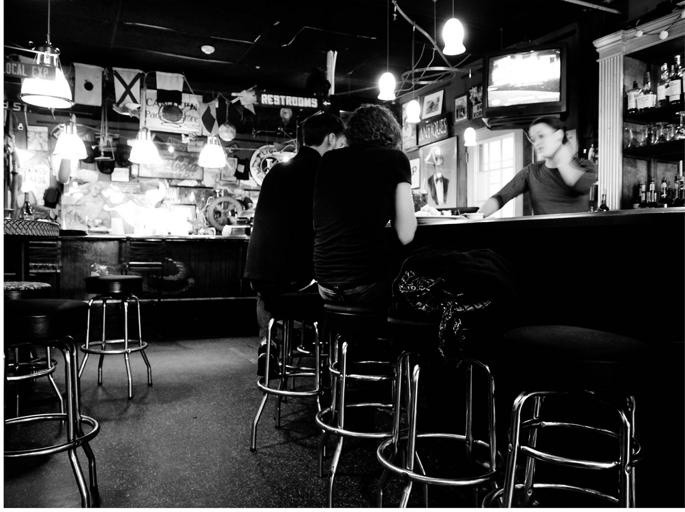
[243,113,345,321]
[477,113,599,218]
[309,103,419,310]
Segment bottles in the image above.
[674,160,685,206]
[627,53,685,113]
[19,191,32,219]
[631,175,670,208]
[587,194,610,213]
[580,143,598,164]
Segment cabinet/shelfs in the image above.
[594,13,685,209]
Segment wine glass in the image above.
[623,110,685,148]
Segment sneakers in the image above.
[257,338,280,378]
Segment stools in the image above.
[4,298,99,508]
[496,323,643,508]
[310,302,398,509]
[249,303,334,479]
[76,275,153,400]
[5,279,64,428]
[376,315,505,508]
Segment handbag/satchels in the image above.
[390,250,513,366]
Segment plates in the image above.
[414,215,459,224]
[220,225,251,238]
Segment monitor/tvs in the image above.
[482,45,568,130]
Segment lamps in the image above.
[439,2,467,55]
[464,72,477,147]
[197,132,226,169]
[92,103,115,160]
[403,32,422,125]
[129,73,162,164]
[21,0,73,110]
[218,99,237,143]
[53,115,87,159]
[376,7,398,100]
